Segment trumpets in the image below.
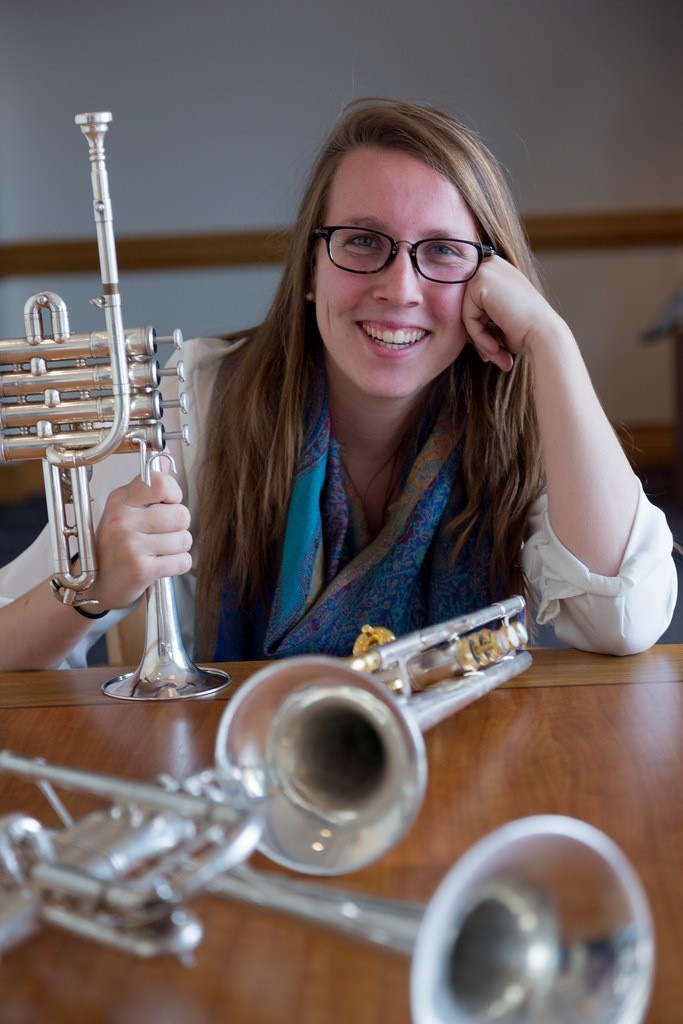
[0,110,235,705]
[0,750,658,1024]
[160,593,535,881]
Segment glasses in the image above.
[312,224,495,284]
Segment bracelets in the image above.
[70,553,110,619]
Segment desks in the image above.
[0,643,683,1024]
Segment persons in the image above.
[0,100,678,675]
